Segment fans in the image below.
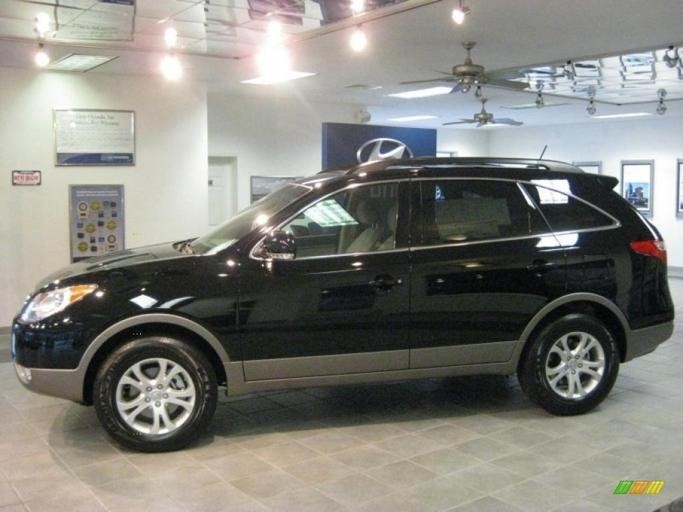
[400,41,530,128]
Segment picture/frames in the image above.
[619,156,655,216]
[51,105,135,263]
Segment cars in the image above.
[10,156,674,452]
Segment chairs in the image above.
[341,199,398,252]
